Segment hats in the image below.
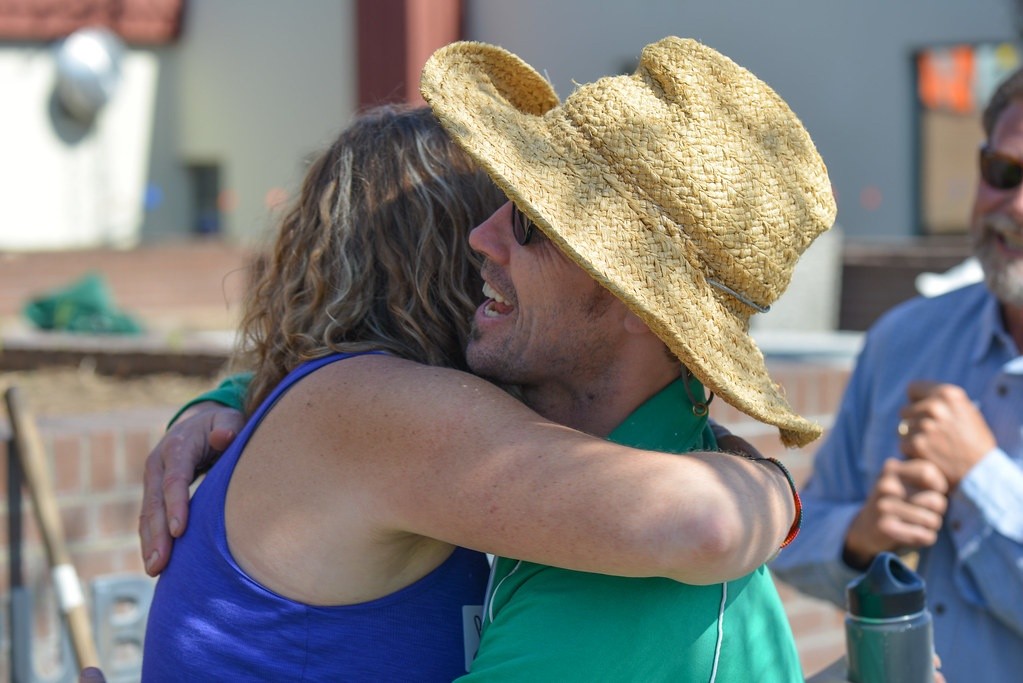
[420,35,838,451]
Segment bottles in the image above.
[844,550,935,683]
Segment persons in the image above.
[139,36,839,683]
[140,104,795,683]
[765,67,1023,683]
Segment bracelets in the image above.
[759,457,803,548]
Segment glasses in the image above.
[511,203,535,244]
[979,147,1023,190]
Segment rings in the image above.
[898,420,909,435]
